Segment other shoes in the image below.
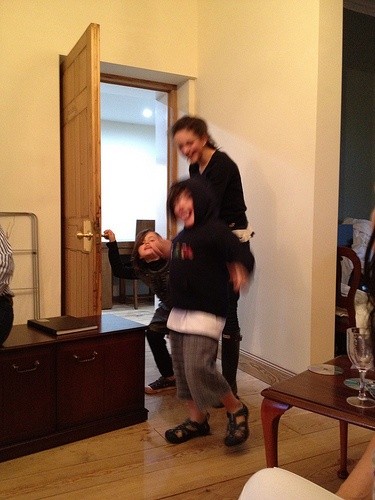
[212,394,240,409]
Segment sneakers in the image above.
[145,374,178,395]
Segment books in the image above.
[28,315,97,335]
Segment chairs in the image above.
[112,220,155,309]
[335,245,361,353]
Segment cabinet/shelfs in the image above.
[1,314,149,463]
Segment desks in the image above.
[260,355,375,479]
[101,241,136,310]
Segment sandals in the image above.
[164,413,211,445]
[223,402,250,447]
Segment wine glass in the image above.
[347,327,375,408]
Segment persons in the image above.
[104,230,176,394]
[151,178,255,446]
[172,116,248,408]
[0,224,14,346]
